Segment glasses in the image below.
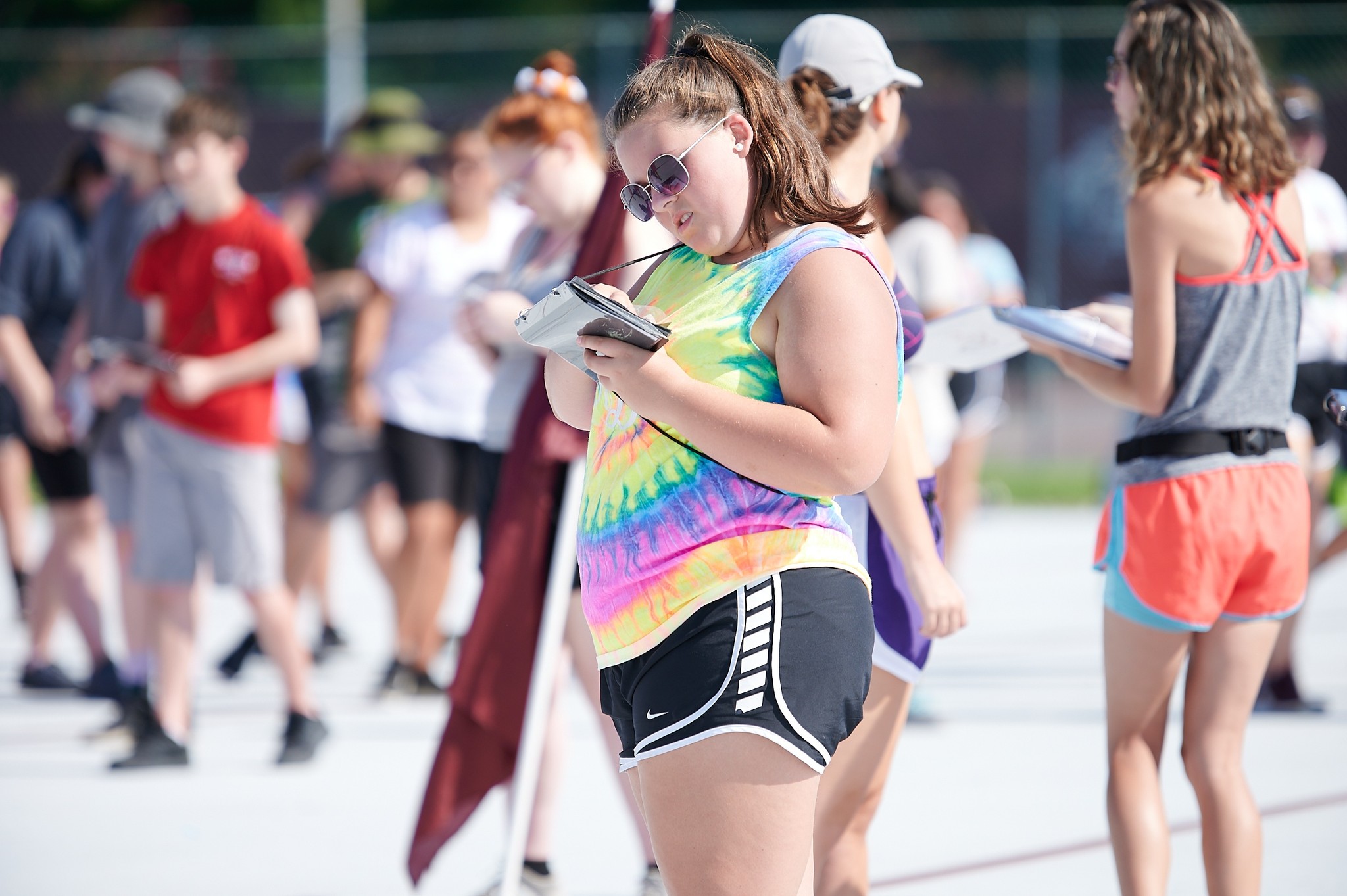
[859,82,907,110]
[1105,56,1125,85]
[620,116,730,222]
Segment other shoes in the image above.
[111,730,187,766]
[277,708,330,766]
[382,660,441,697]
[19,662,76,692]
[79,654,118,696]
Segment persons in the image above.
[1256,86,1347,713]
[773,15,969,895]
[1021,1,1310,896]
[545,23,904,896]
[456,53,674,896]
[0,64,1036,771]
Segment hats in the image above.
[776,14,923,109]
[66,65,183,151]
